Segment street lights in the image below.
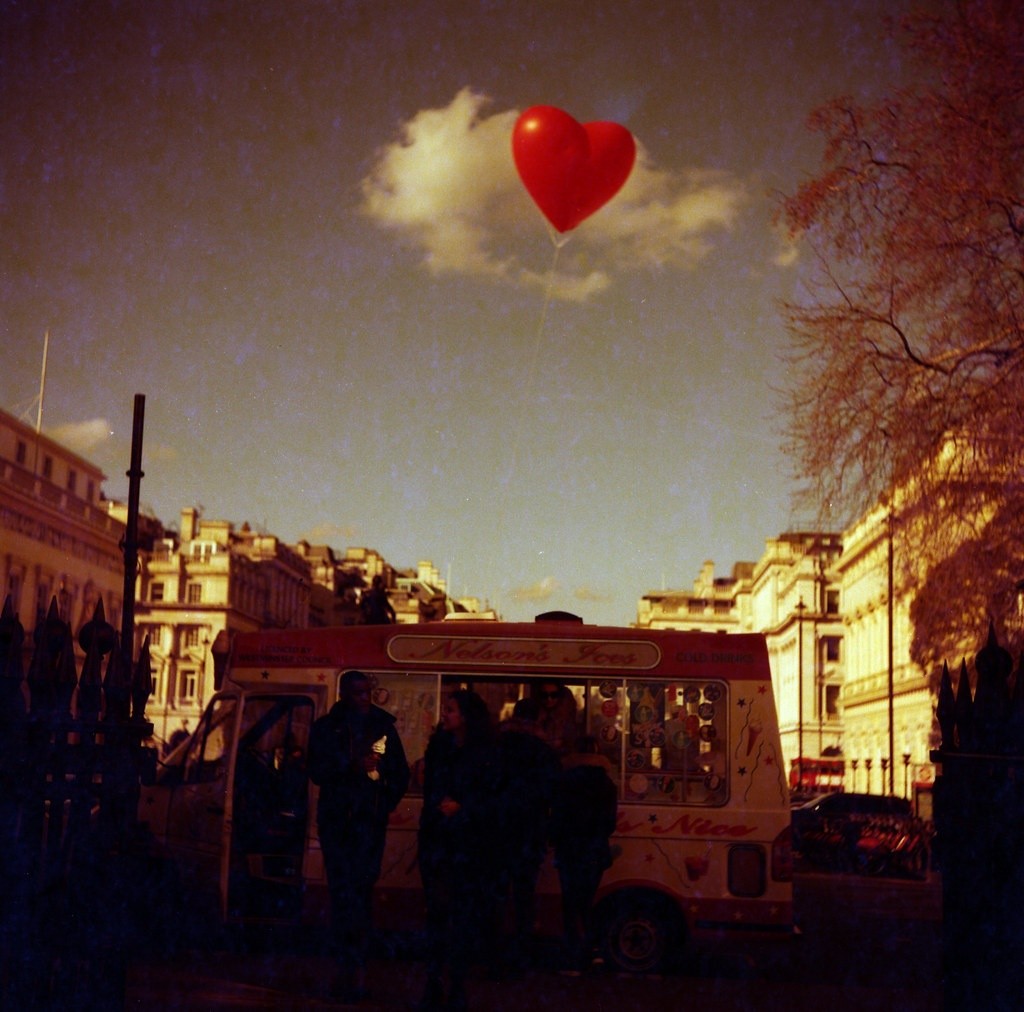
[160,650,174,761]
[199,635,210,722]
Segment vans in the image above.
[139,611,803,977]
[791,792,912,863]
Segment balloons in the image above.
[512,105,638,233]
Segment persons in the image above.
[419,688,618,1012]
[307,669,410,1000]
[169,718,191,748]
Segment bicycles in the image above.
[811,812,933,879]
